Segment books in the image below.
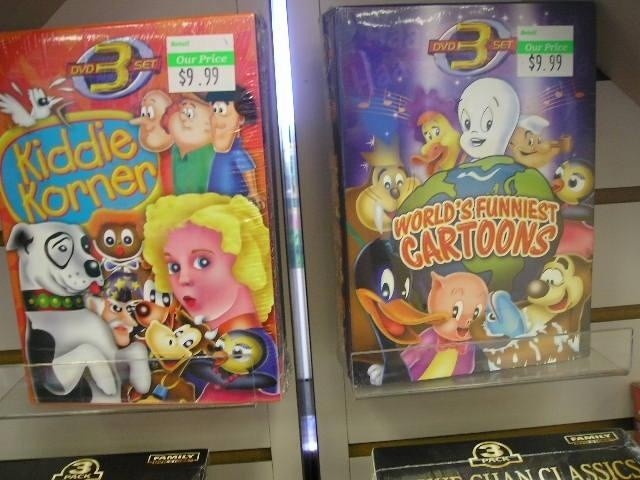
[319,2,598,390]
[0,446,209,477]
[1,12,294,408]
[369,426,640,480]
